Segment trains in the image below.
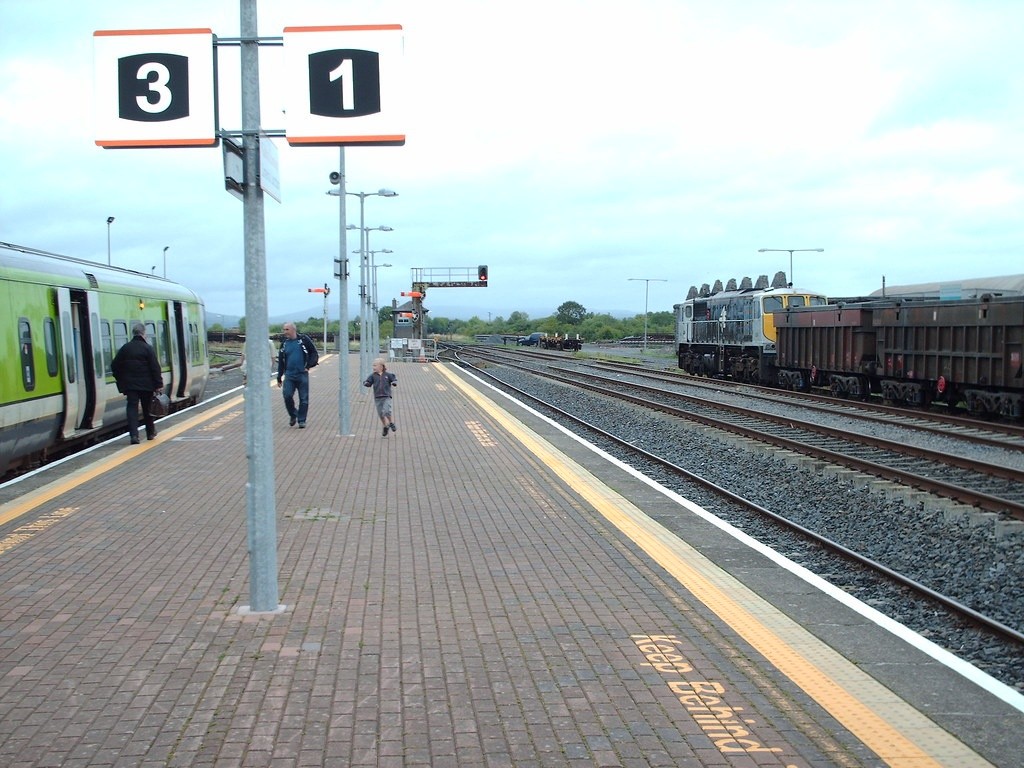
[1,240,210,485]
[207,330,360,343]
[518,332,586,352]
[672,287,1024,426]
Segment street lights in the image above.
[757,247,825,289]
[163,246,171,279]
[358,263,393,360]
[151,265,155,272]
[344,222,394,400]
[107,216,115,265]
[327,187,398,394]
[627,278,668,354]
[349,247,394,375]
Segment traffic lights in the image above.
[480,267,487,280]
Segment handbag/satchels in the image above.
[149,392,170,417]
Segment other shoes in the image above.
[390,423,396,431]
[382,425,390,436]
[147,430,157,440]
[299,421,306,427]
[131,435,140,444]
[289,415,297,426]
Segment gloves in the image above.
[392,383,397,387]
[366,384,371,387]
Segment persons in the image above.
[277,321,319,428]
[539,336,579,351]
[363,358,398,436]
[110,322,164,444]
[502,336,507,345]
[21,331,34,390]
[240,327,277,458]
